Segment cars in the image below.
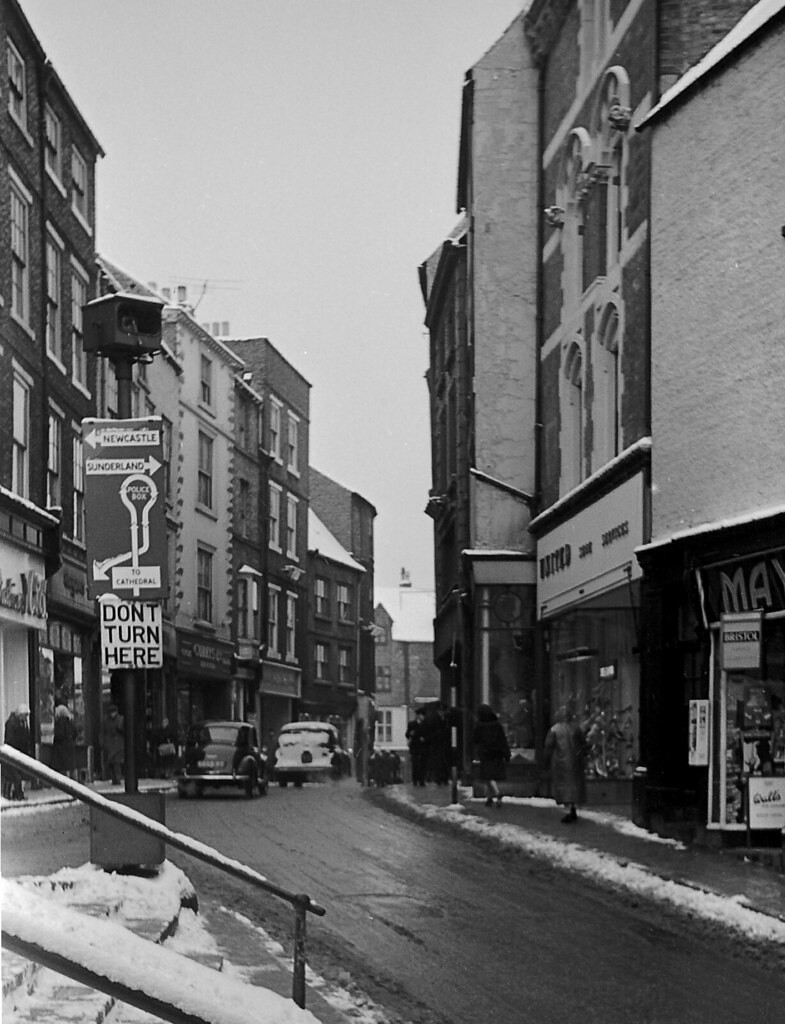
[175,720,270,798]
[274,721,352,787]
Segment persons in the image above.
[746,740,771,772]
[543,707,594,821]
[102,705,126,785]
[4,705,34,800]
[372,703,449,786]
[51,706,78,780]
[150,716,176,780]
[264,729,280,780]
[475,704,511,807]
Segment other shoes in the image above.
[485,800,492,806]
[12,791,27,799]
[496,793,503,807]
[562,808,577,823]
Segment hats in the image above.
[415,708,426,714]
[108,705,118,712]
